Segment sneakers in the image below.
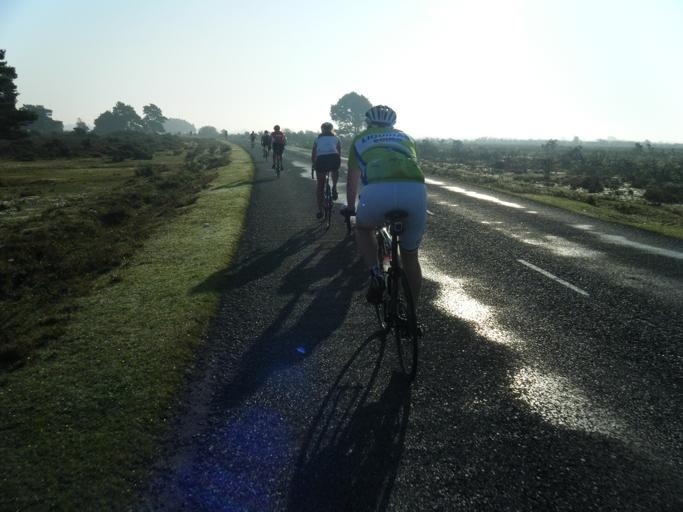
[281,166,283,170]
[412,333,424,349]
[272,164,275,168]
[317,213,323,218]
[370,275,385,289]
[332,189,338,200]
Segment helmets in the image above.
[365,105,396,125]
[321,122,333,130]
[275,125,280,130]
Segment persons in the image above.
[310,122,341,218]
[269,125,286,171]
[342,104,428,341]
[248,130,257,150]
[259,130,270,158]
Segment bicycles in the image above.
[248,135,283,179]
[310,163,337,228]
[342,198,422,381]
